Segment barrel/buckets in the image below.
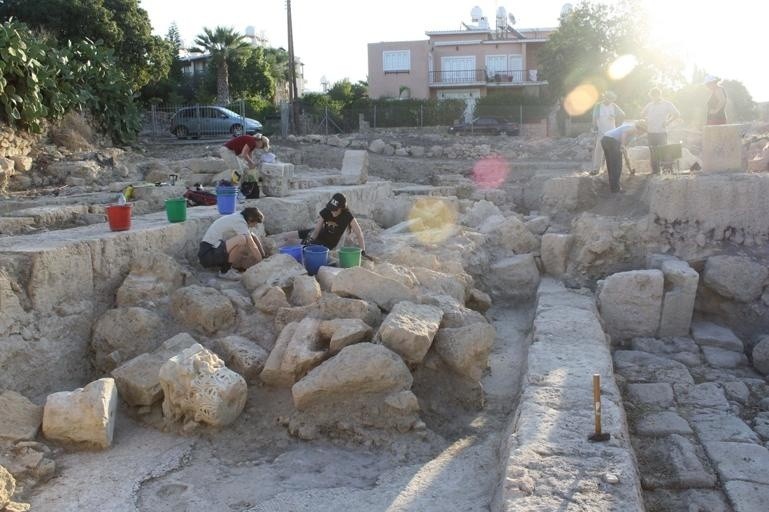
[106,204,133,231]
[279,245,303,263]
[304,246,329,274]
[217,186,238,214]
[339,247,362,267]
[164,198,188,223]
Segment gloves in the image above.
[362,251,373,260]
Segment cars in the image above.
[170,104,267,142]
[449,114,522,139]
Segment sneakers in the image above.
[590,170,599,175]
[218,269,240,281]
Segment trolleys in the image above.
[646,142,687,176]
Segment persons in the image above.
[196,206,265,282]
[589,90,628,177]
[600,119,645,194]
[640,89,679,173]
[265,193,374,261]
[218,133,272,186]
[702,74,728,124]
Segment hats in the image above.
[325,193,346,211]
[703,76,720,86]
[634,119,647,131]
[605,91,617,101]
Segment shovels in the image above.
[623,152,635,176]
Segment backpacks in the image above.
[184,189,216,206]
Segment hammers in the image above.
[588,374,610,443]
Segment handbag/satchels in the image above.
[241,182,258,198]
